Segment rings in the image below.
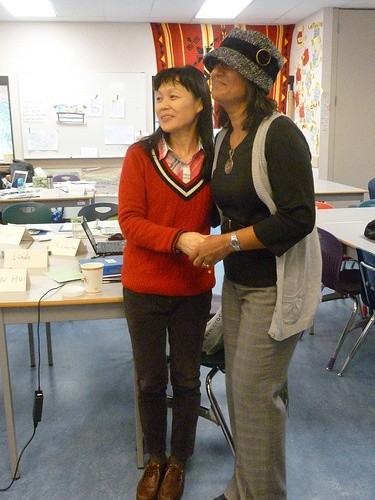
[202,262,209,269]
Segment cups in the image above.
[18,178,24,193]
[47,175,53,189]
[71,216,83,237]
[81,263,104,294]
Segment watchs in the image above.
[229,231,241,251]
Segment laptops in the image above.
[2,171,28,193]
[81,215,127,255]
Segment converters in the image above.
[33,390,44,422]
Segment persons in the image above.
[117,64,222,500]
[200,25,323,500]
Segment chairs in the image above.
[2,203,54,225]
[160,310,237,460]
[306,225,367,371]
[78,202,119,221]
[337,251,375,380]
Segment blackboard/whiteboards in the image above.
[17,72,148,160]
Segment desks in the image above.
[313,177,371,206]
[0,180,97,219]
[0,221,149,480]
[312,206,375,257]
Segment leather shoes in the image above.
[136,459,168,500]
[158,458,186,500]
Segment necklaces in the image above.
[224,128,249,174]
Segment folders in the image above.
[78,255,123,276]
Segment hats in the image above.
[203,27,285,94]
